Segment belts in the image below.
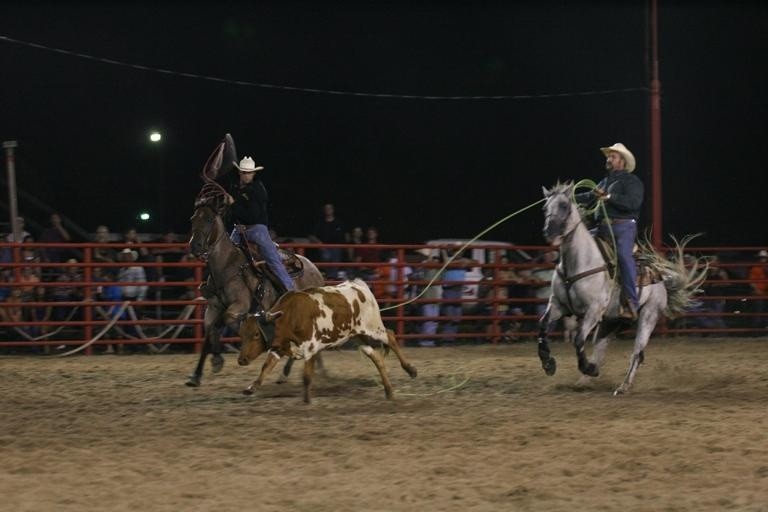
[601,218,635,226]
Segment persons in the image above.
[678,250,768,338]
[213,155,294,295]
[574,142,645,319]
[271,206,537,347]
[3,212,241,355]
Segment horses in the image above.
[537,176,719,397]
[185,193,329,388]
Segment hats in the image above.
[600,142,636,173]
[232,156,264,172]
[118,249,138,262]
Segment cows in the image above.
[226,277,417,404]
